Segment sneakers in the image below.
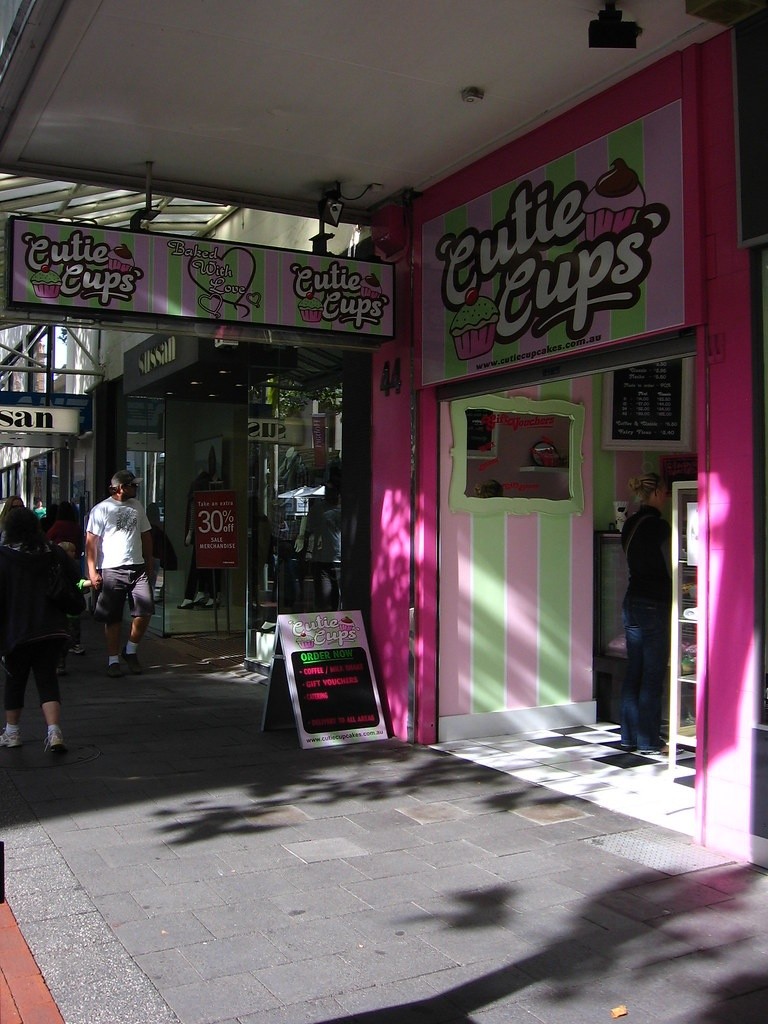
[0,728,22,747]
[43,729,66,753]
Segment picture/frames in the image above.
[464,408,498,460]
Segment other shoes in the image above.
[120,647,144,675]
[620,742,636,752]
[68,644,85,655]
[56,665,69,673]
[640,746,683,756]
[107,662,121,678]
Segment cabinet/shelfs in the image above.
[669,480,698,783]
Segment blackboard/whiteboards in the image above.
[276,610,390,750]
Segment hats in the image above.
[110,470,143,488]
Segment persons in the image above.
[178,471,219,609]
[86,470,156,677]
[619,473,672,752]
[0,496,100,753]
[310,485,341,611]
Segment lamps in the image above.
[317,180,344,227]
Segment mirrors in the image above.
[448,395,585,516]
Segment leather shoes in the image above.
[176,602,194,610]
[194,598,207,606]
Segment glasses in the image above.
[11,504,24,510]
[127,484,139,489]
